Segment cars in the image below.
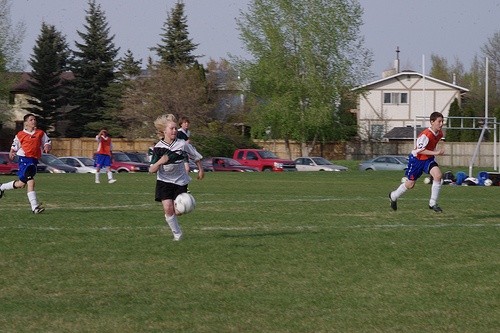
[36,153,77,173]
[33,153,59,175]
[0,152,22,176]
[146,148,213,173]
[359,156,412,171]
[49,156,109,176]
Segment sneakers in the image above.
[32,207,45,214]
[95,180,100,183]
[109,179,116,184]
[173,232,184,241]
[388,192,398,212]
[0,183,4,200]
[428,204,442,213]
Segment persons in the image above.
[388,111,446,213]
[148,113,204,242]
[174,116,193,194]
[94,128,117,184]
[0,114,53,214]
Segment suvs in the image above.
[233,149,297,173]
[91,150,150,173]
[293,156,348,174]
[202,157,258,173]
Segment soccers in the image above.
[175,192,196,214]
[400,176,408,183]
[423,177,432,184]
[484,178,493,186]
